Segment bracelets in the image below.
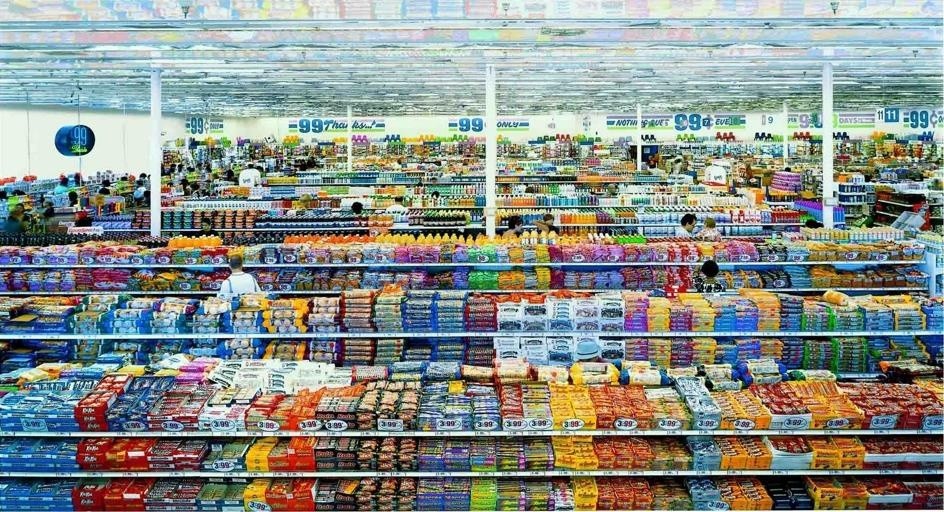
[693,270,699,275]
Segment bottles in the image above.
[137,234,169,248]
[800,225,905,240]
[552,208,637,224]
[407,209,471,226]
[521,227,589,244]
[255,214,394,229]
[171,234,222,249]
[383,210,407,222]
[493,209,545,225]
[915,231,944,268]
[588,231,646,244]
[471,207,484,223]
[220,234,520,247]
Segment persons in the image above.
[675,213,697,238]
[693,260,726,293]
[501,215,523,235]
[417,182,423,187]
[220,254,262,294]
[431,177,437,183]
[695,218,721,239]
[1,153,319,228]
[805,219,820,228]
[350,202,363,214]
[385,196,408,213]
[431,191,439,199]
[537,214,559,235]
[604,183,616,197]
